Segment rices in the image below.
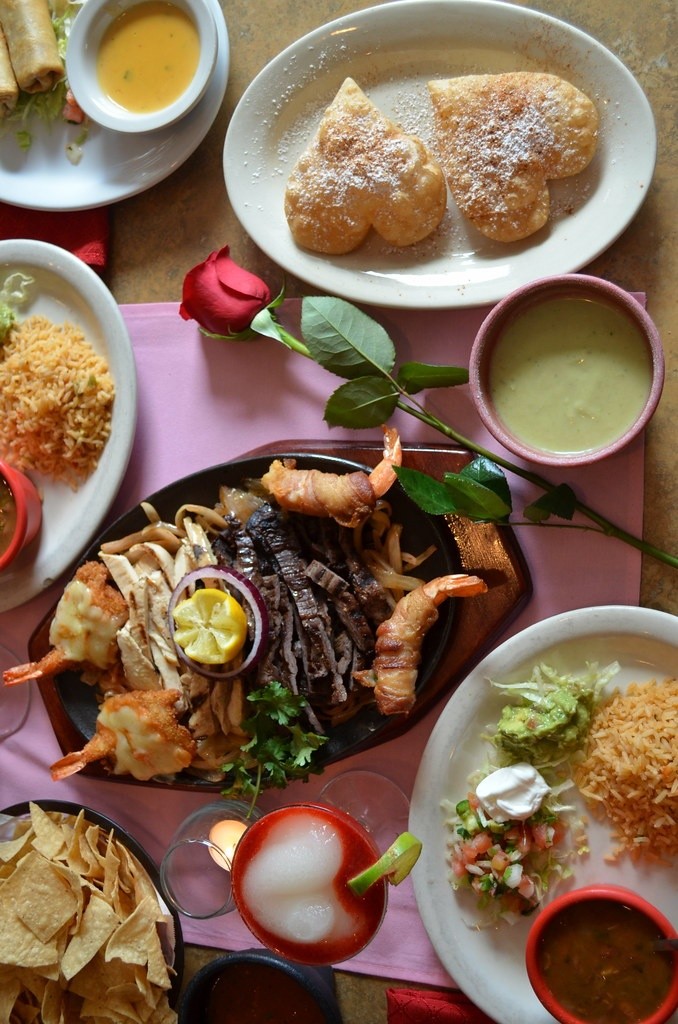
[579,673,678,869]
[0,315,115,491]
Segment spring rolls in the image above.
[0,0,63,118]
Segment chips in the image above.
[0,798,179,1023]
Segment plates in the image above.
[221,1,658,310]
[406,604,678,1024]
[0,0,233,213]
[1,238,139,618]
[0,799,182,1024]
[23,438,537,793]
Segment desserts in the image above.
[284,73,598,254]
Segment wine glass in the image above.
[229,771,410,967]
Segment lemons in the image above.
[173,589,246,666]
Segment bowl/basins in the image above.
[524,887,678,1024]
[467,276,666,468]
[63,0,218,136]
[0,462,39,572]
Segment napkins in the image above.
[0,288,652,990]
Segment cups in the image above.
[159,796,263,922]
[171,948,344,1024]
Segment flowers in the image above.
[166,244,677,559]
